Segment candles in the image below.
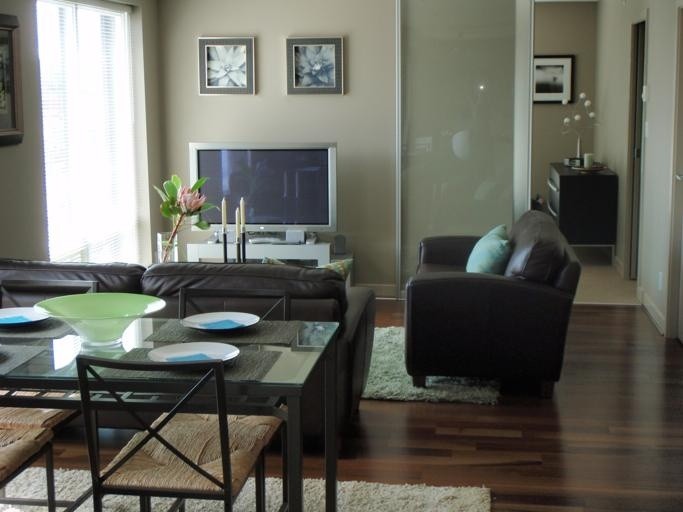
[221,197,227,233]
[240,196,246,232]
[235,207,241,244]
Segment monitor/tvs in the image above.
[188,141,336,245]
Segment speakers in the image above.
[334,235,345,255]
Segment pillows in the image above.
[262,256,355,282]
[466,223,511,276]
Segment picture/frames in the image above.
[197,36,257,96]
[285,36,345,95]
[532,54,576,105]
[0,13,25,146]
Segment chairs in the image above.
[74,352,289,511]
[0,275,100,484]
[1,424,54,511]
[176,289,292,500]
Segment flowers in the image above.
[153,176,219,264]
[560,92,601,138]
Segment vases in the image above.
[575,136,584,163]
[157,233,181,264]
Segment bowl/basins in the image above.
[39,291,167,344]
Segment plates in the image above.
[181,312,262,332]
[0,306,49,324]
[149,340,239,365]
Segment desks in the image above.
[185,236,340,270]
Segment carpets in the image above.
[1,467,492,512]
[360,325,500,404]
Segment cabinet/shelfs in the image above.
[547,162,618,265]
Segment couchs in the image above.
[4,258,377,454]
[405,210,581,401]
